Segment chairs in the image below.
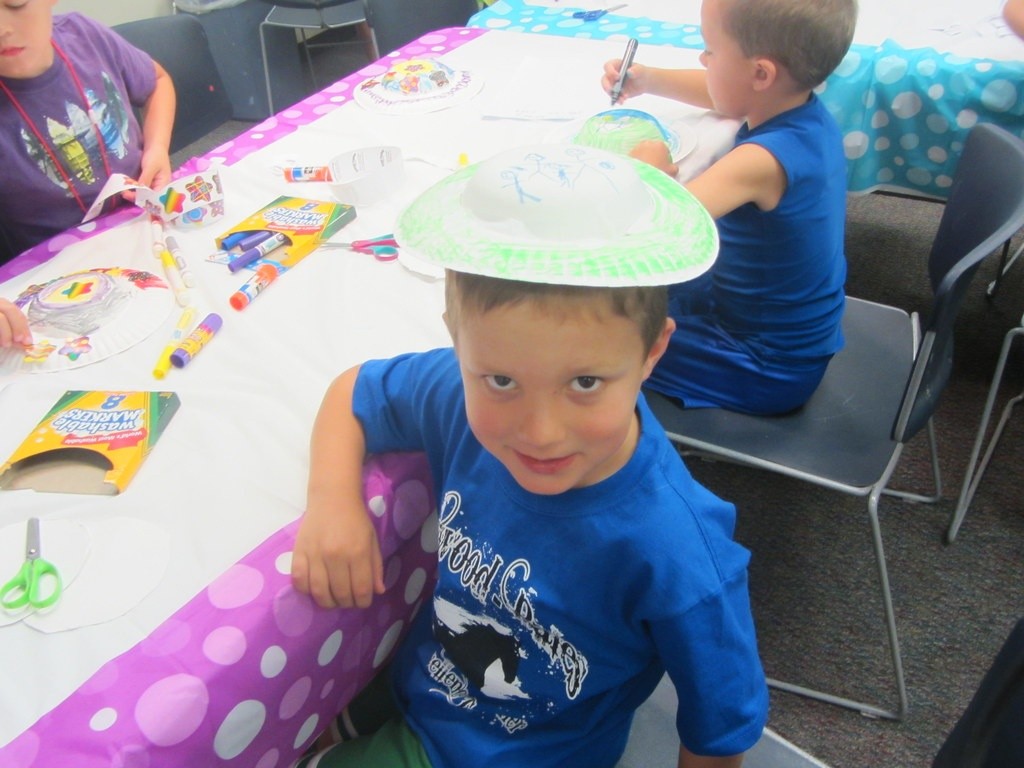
[941,231,1024,548]
[642,122,1024,723]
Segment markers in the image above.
[283,166,333,183]
[222,231,289,311]
[150,213,197,308]
[611,38,639,106]
[154,311,224,379]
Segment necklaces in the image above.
[0,38,116,214]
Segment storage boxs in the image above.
[0,390,182,495]
[213,195,357,269]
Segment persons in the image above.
[0,0,175,351]
[603,0,857,416]
[293,266,768,768]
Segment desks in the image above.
[0,26,745,768]
[466,0,1024,203]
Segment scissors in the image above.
[1,516,63,609]
[324,233,400,262]
